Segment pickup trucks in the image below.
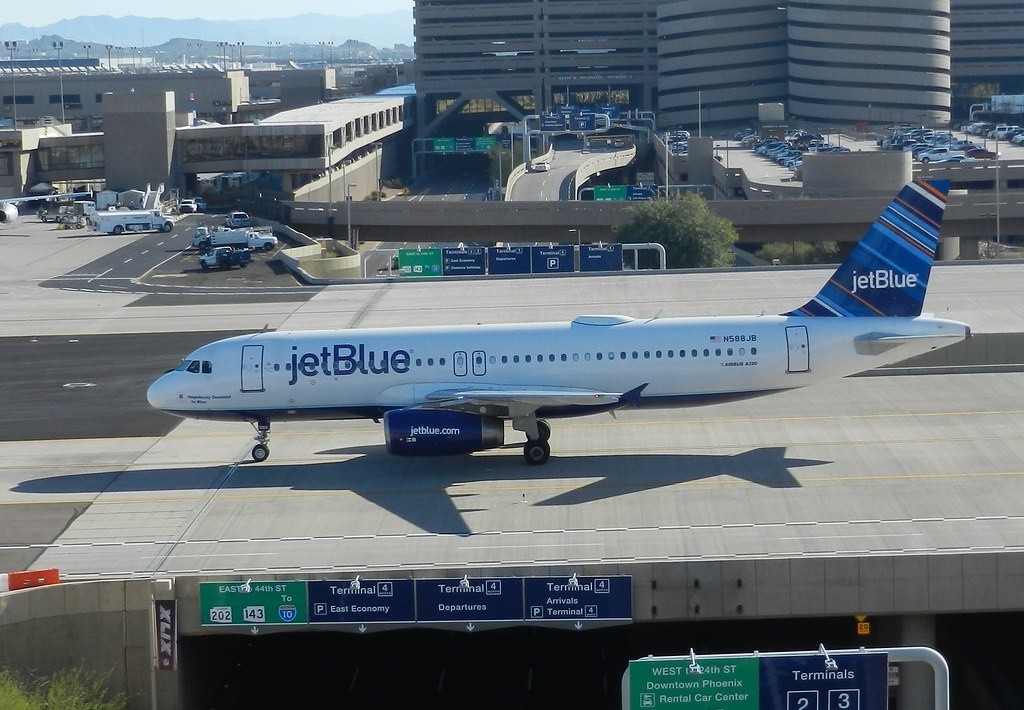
[199,246,251,269]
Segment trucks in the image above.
[191,226,278,252]
[91,208,175,234]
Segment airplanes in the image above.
[147,178,976,467]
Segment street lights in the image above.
[83,44,91,76]
[318,40,334,69]
[104,45,138,71]
[267,40,281,70]
[4,40,20,131]
[52,41,65,125]
[217,41,244,78]
[347,183,357,249]
[327,145,335,217]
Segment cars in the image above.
[533,162,550,170]
[179,196,208,213]
[733,124,852,168]
[959,121,1024,143]
[225,211,253,228]
[876,127,1002,163]
[662,130,690,156]
